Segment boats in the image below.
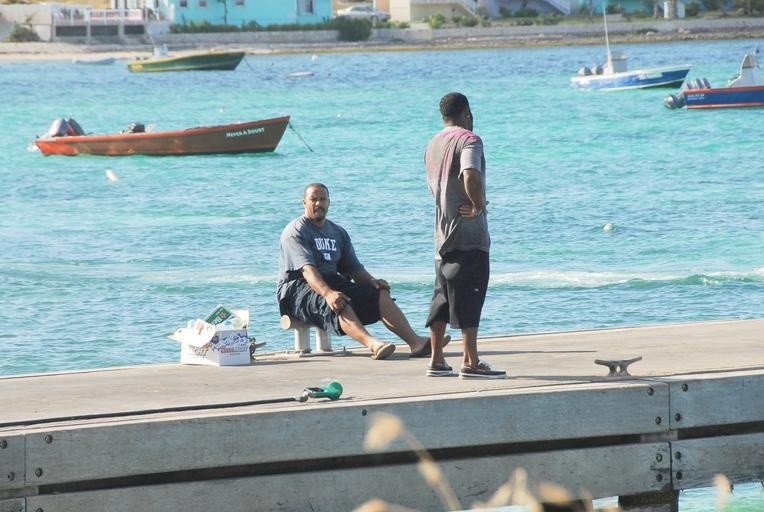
[75,55,117,66]
[33,114,290,157]
[660,48,764,111]
[567,1,692,93]
[128,43,243,73]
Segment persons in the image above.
[273,183,449,362]
[422,93,509,380]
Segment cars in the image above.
[334,3,392,24]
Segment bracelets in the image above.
[472,209,483,218]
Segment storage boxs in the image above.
[168,305,251,366]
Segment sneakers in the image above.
[426,359,453,376]
[458,359,505,379]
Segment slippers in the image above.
[409,334,450,358]
[370,343,394,360]
